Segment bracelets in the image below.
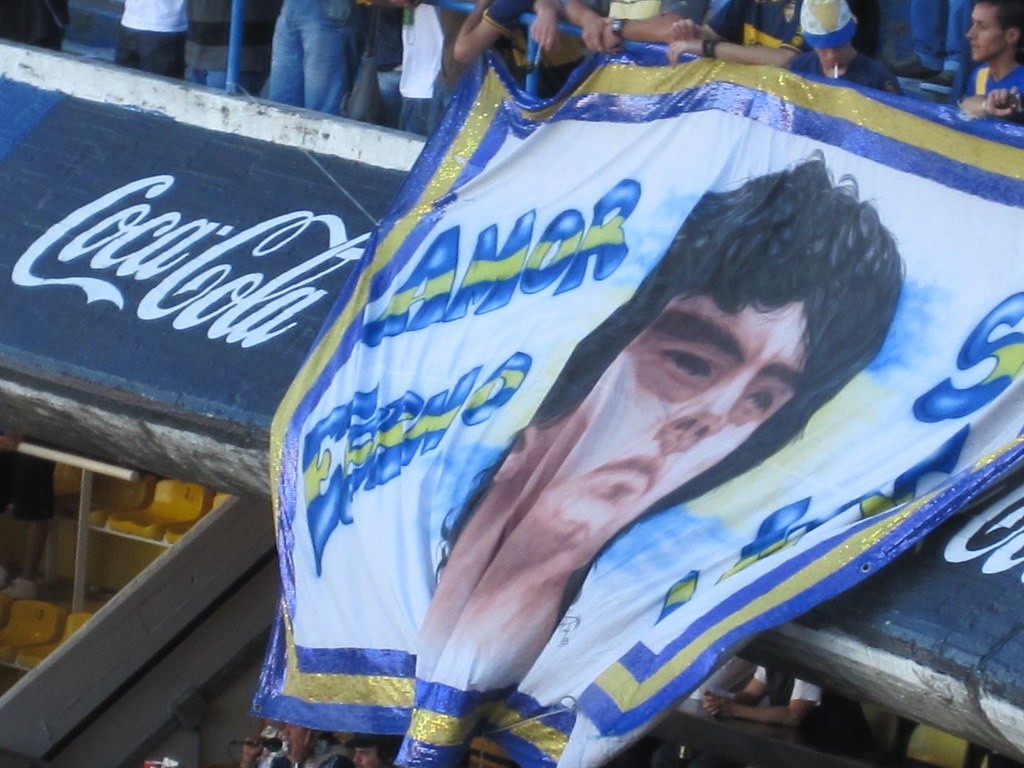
[982,99,988,112]
[703,39,728,58]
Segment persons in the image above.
[239,664,823,768]
[0,426,57,600]
[0,0,1024,137]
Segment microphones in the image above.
[239,738,282,753]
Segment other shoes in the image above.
[920,68,957,96]
[892,55,940,84]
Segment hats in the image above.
[800,0,858,48]
[345,734,395,748]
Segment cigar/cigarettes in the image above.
[834,62,839,79]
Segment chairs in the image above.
[908,723,989,768]
[1,439,235,667]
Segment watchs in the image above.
[611,18,626,41]
